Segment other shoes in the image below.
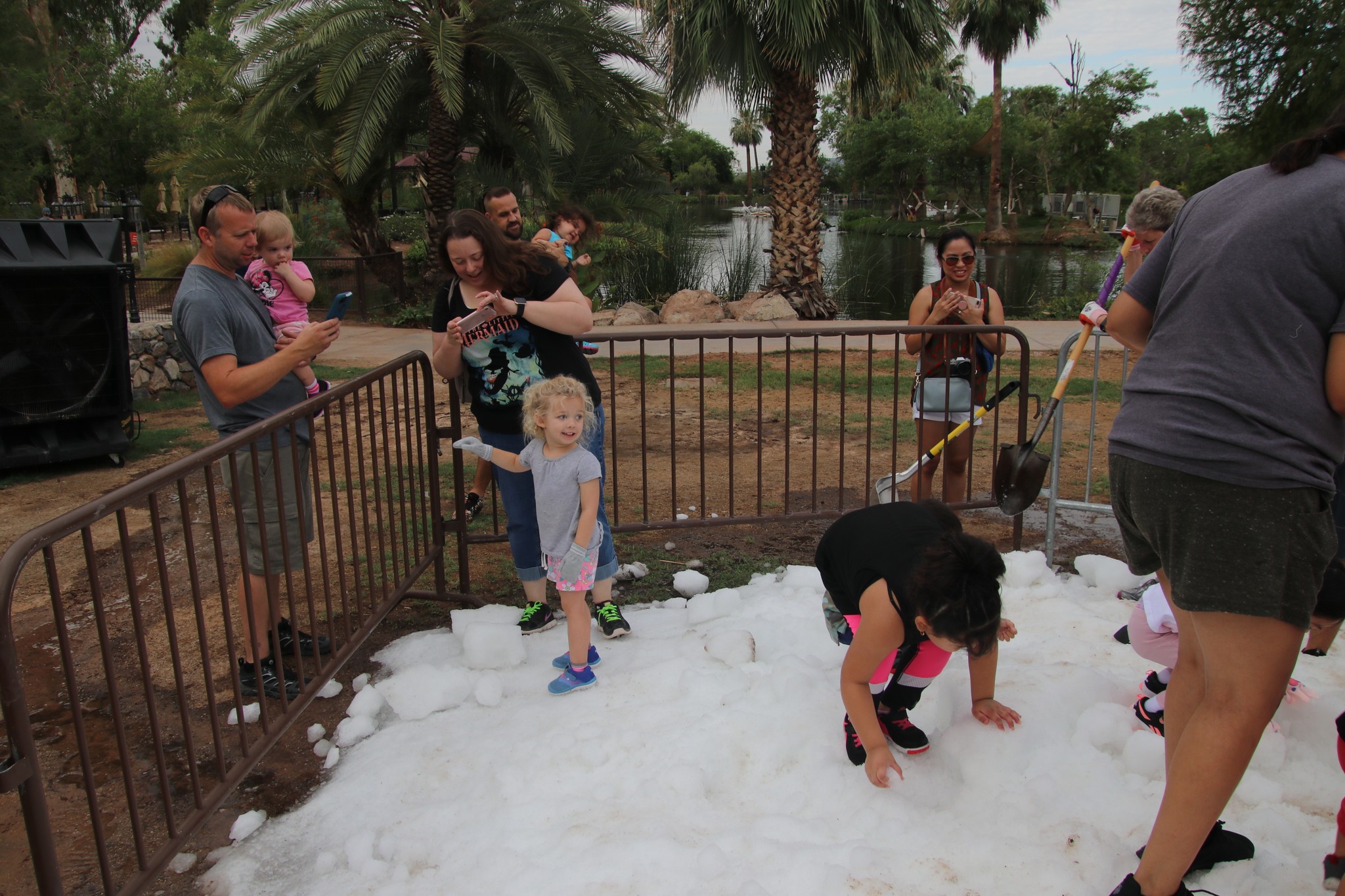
[1137,819,1254,873]
[1109,873,1219,896]
[1323,855,1345,890]
[307,380,331,420]
[575,340,599,355]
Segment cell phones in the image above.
[325,292,353,321]
[945,292,981,309]
[457,306,497,333]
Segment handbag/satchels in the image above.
[909,373,971,412]
[974,280,995,373]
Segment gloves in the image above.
[558,542,587,584]
[1079,301,1109,327]
[1121,224,1141,250]
[453,437,494,461]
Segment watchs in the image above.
[564,259,573,273]
[513,298,527,318]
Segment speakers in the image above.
[0,217,134,466]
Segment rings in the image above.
[494,293,498,301]
[958,310,961,314]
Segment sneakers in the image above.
[1132,693,1166,737]
[878,705,930,754]
[451,491,485,526]
[238,654,313,700]
[553,645,602,670]
[844,713,866,766]
[515,600,557,634]
[548,662,598,695]
[594,599,631,639]
[268,616,332,656]
[1139,669,1168,698]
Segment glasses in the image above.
[941,250,975,267]
[200,184,240,227]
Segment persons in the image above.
[1105,119,1345,896]
[1078,186,1186,602]
[431,209,631,639]
[453,187,577,524]
[531,202,599,355]
[244,210,331,419]
[815,501,1022,788]
[905,228,1006,514]
[1127,559,1345,737]
[452,376,602,695]
[171,185,330,700]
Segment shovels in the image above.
[994,179,1161,516]
[870,381,1018,507]
